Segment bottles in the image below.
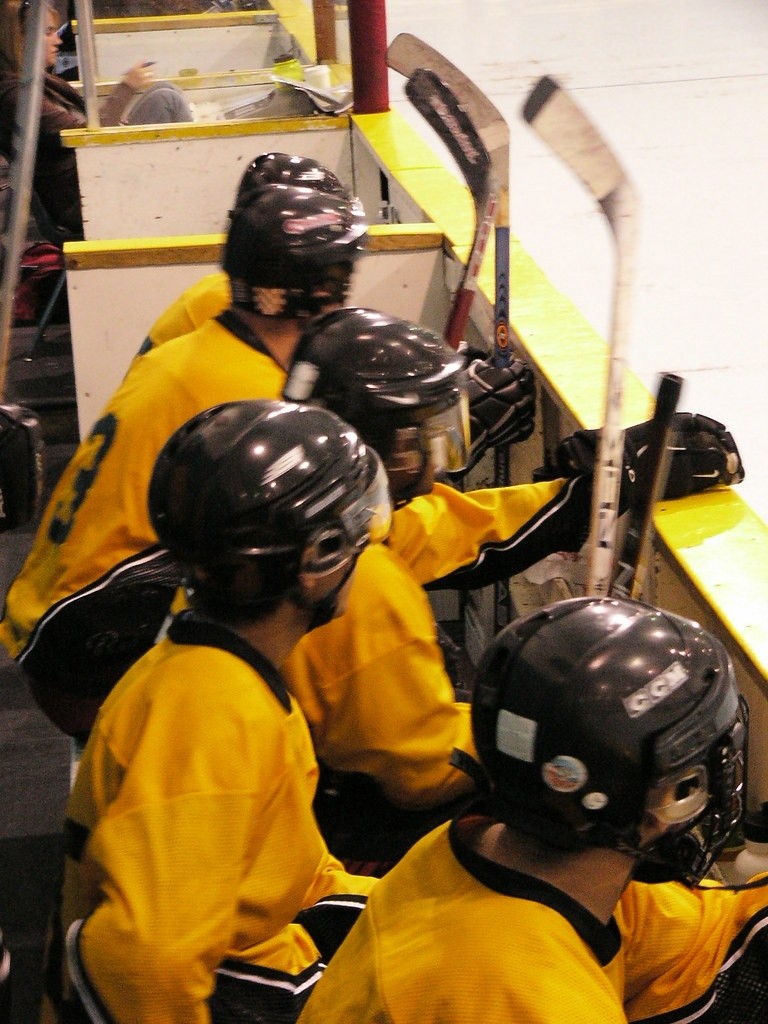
[272,52,303,91]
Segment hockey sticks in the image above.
[402,68,501,351]
[384,31,513,635]
[519,73,645,601]
[607,370,686,605]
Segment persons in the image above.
[154,305,745,878]
[0,1,535,663]
[294,596,768,1024]
[45,400,383,1024]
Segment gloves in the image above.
[447,343,537,482]
[558,412,745,517]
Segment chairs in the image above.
[0,136,83,363]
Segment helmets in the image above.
[150,400,386,608]
[288,304,462,461]
[474,599,749,885]
[238,153,349,202]
[223,183,360,301]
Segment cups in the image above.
[304,63,330,92]
